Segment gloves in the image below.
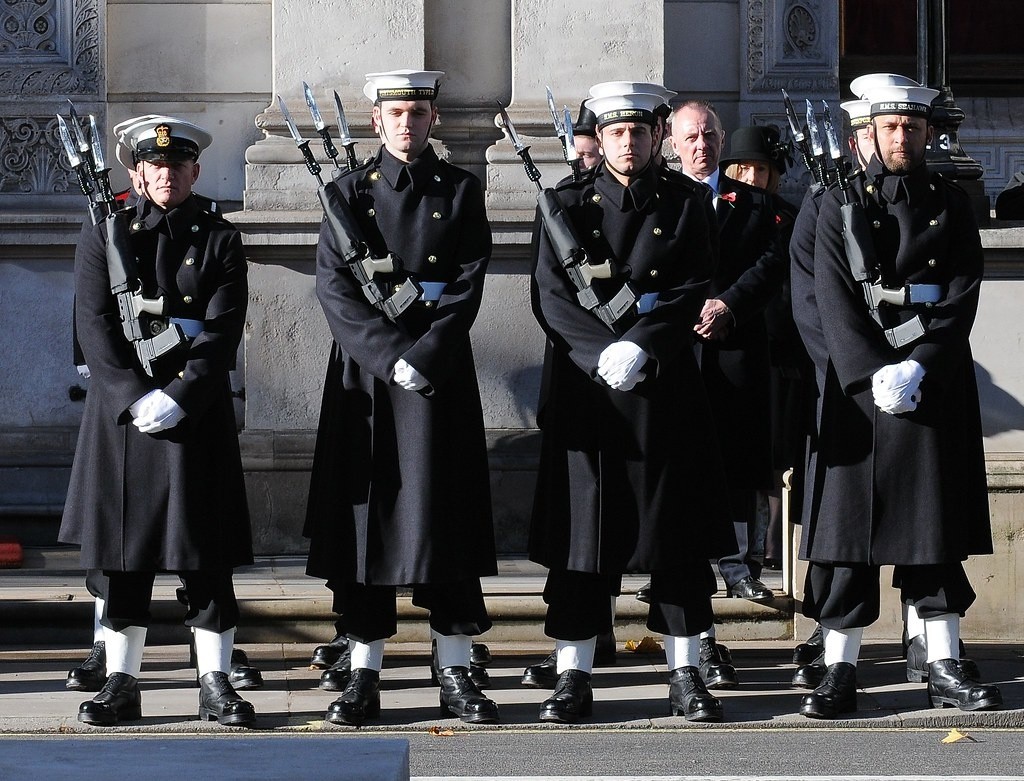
[127,388,188,434]
[76,365,91,379]
[597,342,648,392]
[392,358,428,390]
[870,360,927,415]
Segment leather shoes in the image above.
[66,558,1002,723]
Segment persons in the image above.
[520,81,799,724]
[789,73,1002,717]
[302,71,502,724]
[57,114,264,726]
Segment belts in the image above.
[140,316,205,338]
[884,284,943,306]
[622,293,659,314]
[387,281,449,300]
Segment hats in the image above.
[362,70,445,107]
[850,73,921,101]
[573,98,596,137]
[113,115,213,171]
[867,86,940,119]
[719,126,785,168]
[840,100,873,131]
[583,80,678,131]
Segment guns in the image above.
[55,97,186,382]
[301,81,348,178]
[495,97,639,339]
[545,86,586,183]
[781,88,927,368]
[333,90,360,171]
[276,91,425,332]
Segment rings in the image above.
[708,324,712,328]
